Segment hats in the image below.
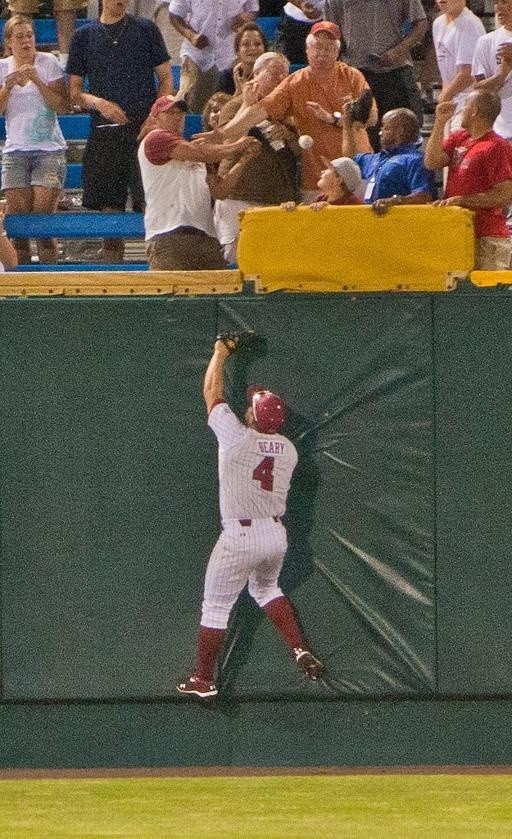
[320,154,362,194]
[150,95,189,117]
[311,21,341,40]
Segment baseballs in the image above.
[298,134,314,149]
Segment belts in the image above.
[238,517,279,526]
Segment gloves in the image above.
[215,331,243,356]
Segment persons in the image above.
[176,331,326,699]
[2,2,511,270]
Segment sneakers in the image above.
[176,677,219,698]
[291,646,326,681]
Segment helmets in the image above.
[247,384,286,433]
